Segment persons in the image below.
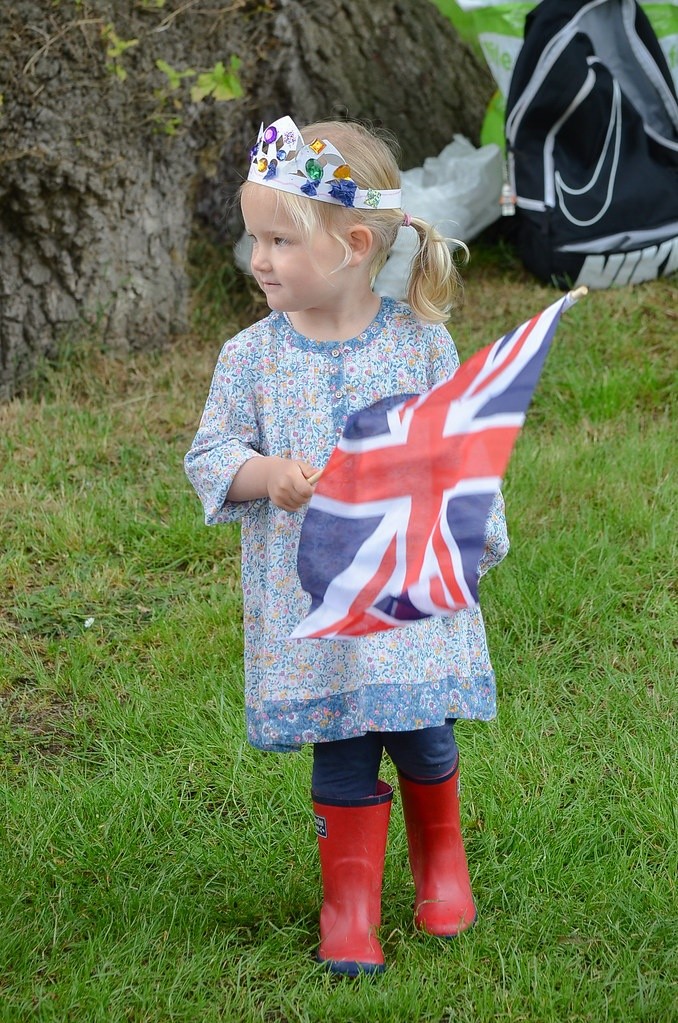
[185,117,509,974]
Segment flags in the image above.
[287,291,576,645]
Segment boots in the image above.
[309,779,393,975]
[397,755,477,942]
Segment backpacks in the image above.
[504,0,678,287]
